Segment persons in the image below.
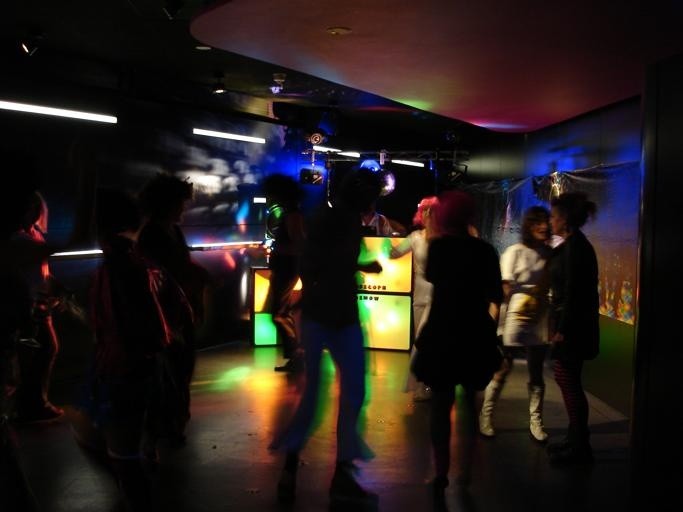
[550,192,601,465]
[478,206,553,442]
[410,191,502,488]
[389,196,441,401]
[74,197,194,456]
[268,169,382,505]
[260,174,306,372]
[136,172,198,448]
[361,201,407,237]
[1,186,65,420]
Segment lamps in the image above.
[210,73,228,95]
[266,80,284,94]
[15,30,47,58]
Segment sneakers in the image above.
[276,471,296,503]
[25,401,63,418]
[274,355,303,372]
[433,475,448,486]
[329,467,378,502]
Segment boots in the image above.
[528,382,548,442]
[477,378,504,437]
[547,425,593,472]
